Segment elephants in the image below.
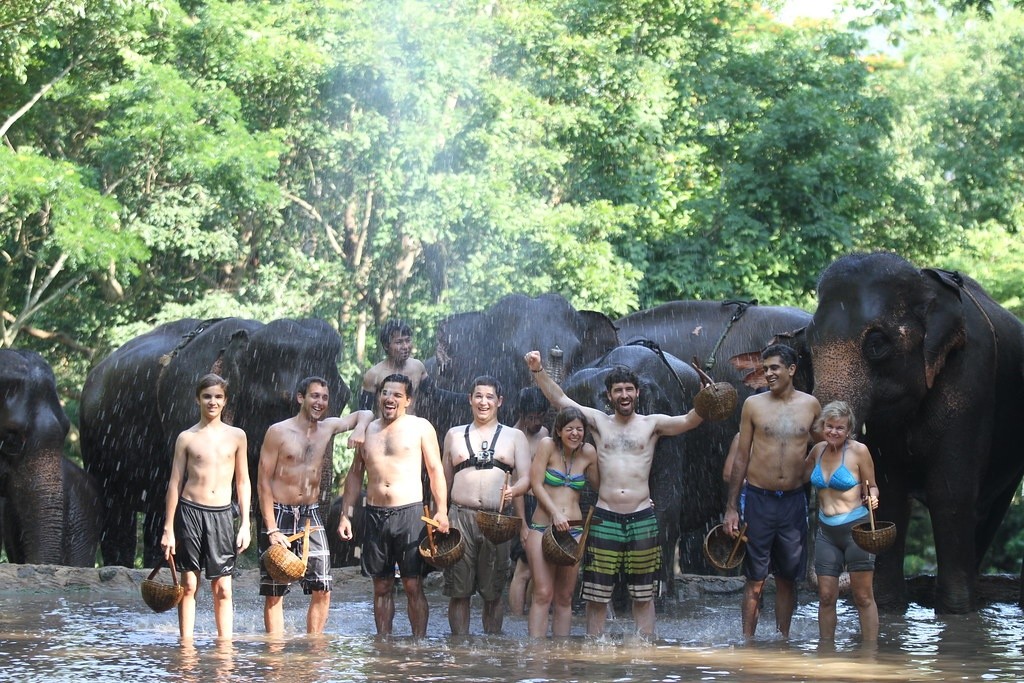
[423,291,618,454]
[521,344,736,609]
[80,315,349,566]
[733,253,1024,608]
[613,304,819,435]
[0,349,99,564]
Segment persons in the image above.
[337,374,450,636]
[722,345,825,642]
[160,373,251,646]
[357,319,472,417]
[723,385,748,525]
[524,350,711,640]
[803,401,879,651]
[524,406,654,636]
[509,386,550,618]
[258,376,375,637]
[441,374,532,635]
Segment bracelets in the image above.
[531,367,545,373]
[266,528,280,535]
